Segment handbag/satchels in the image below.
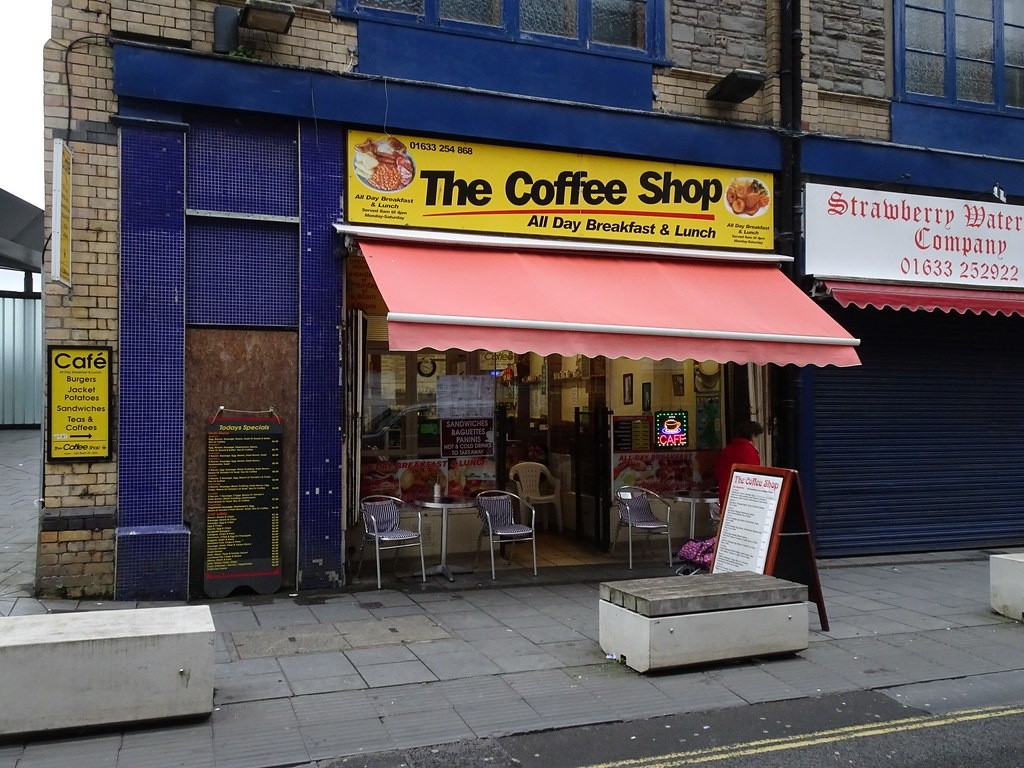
[676,535,716,570]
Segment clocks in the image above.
[418,357,437,377]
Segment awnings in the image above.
[358,240,861,367]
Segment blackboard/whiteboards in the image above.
[440,416,495,458]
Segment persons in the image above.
[713,421,765,516]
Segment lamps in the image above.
[706,69,768,104]
[238,0,296,34]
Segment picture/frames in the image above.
[623,373,634,405]
[672,374,684,396]
[642,382,651,412]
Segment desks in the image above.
[669,489,719,541]
[414,496,476,582]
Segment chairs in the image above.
[474,490,537,580]
[509,462,562,537]
[356,495,426,590]
[610,486,673,570]
